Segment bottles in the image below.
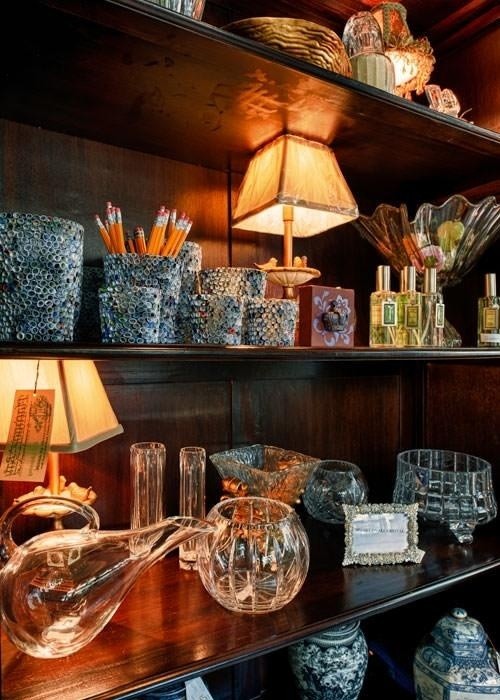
[1,496,215,660]
[369,264,499,349]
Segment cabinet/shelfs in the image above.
[0,0,499,699]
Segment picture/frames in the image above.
[341,503,425,570]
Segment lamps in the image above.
[371,3,436,101]
[230,134,358,299]
[0,359,124,529]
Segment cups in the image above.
[302,460,369,524]
[196,496,311,616]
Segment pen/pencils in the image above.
[94,201,193,257]
